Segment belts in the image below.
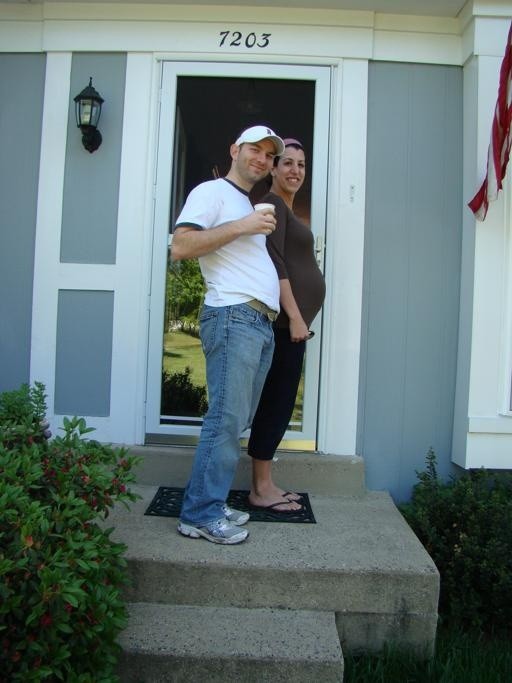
[243,299,279,322]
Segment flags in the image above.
[467,21,511,226]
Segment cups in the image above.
[254,203,275,234]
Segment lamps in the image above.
[72,74,106,153]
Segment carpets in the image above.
[142,485,319,527]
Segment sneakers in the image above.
[177,517,250,546]
[221,505,251,526]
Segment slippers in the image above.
[246,491,307,516]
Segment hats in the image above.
[235,126,285,157]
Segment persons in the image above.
[238,134,329,515]
[165,123,259,548]
